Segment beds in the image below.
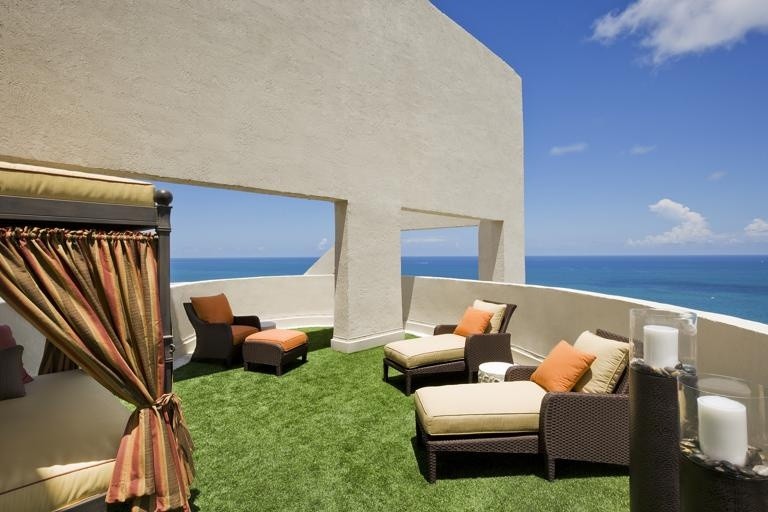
[0,368,133,511]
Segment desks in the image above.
[476,357,514,383]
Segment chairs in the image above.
[382,298,518,397]
[414,327,643,485]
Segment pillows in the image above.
[0,344,26,400]
[190,292,234,324]
[452,305,494,338]
[0,324,35,384]
[529,339,598,393]
[473,299,508,333]
[574,330,630,393]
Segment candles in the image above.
[643,325,679,370]
[696,395,749,469]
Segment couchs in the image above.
[181,301,264,370]
[243,328,310,376]
